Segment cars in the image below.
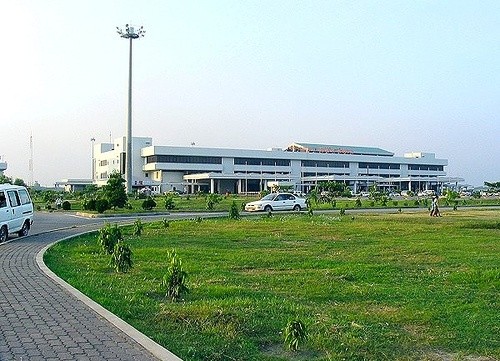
[349,190,436,199]
[244,192,308,213]
[460,190,500,197]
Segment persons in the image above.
[453,197,459,211]
[55,196,62,210]
[429,196,441,217]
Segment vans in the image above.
[0,183,34,243]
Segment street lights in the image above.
[117,23,147,194]
